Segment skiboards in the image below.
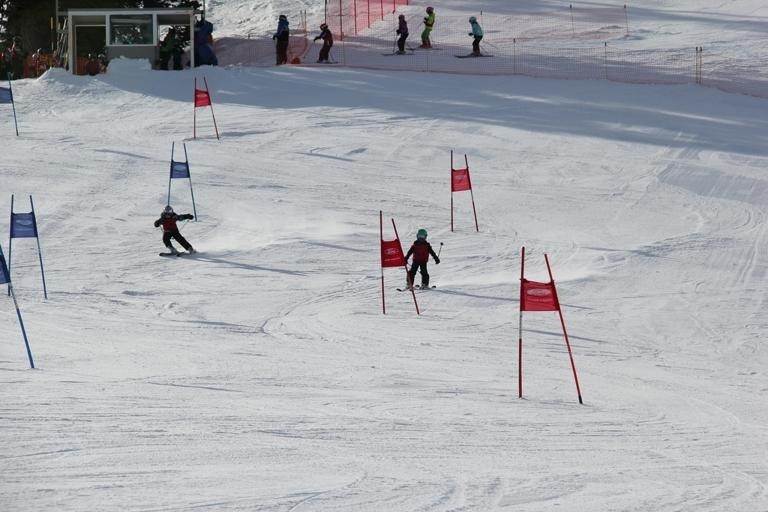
[159,252,201,256]
[381,52,415,56]
[397,286,436,291]
[453,54,493,57]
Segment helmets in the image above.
[417,229,427,239]
[163,206,173,216]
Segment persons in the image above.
[160,27,183,71]
[8,35,28,82]
[314,24,333,63]
[467,16,484,57]
[395,14,409,55]
[272,15,289,65]
[404,229,439,288]
[154,206,196,255]
[84,52,99,74]
[419,7,436,49]
